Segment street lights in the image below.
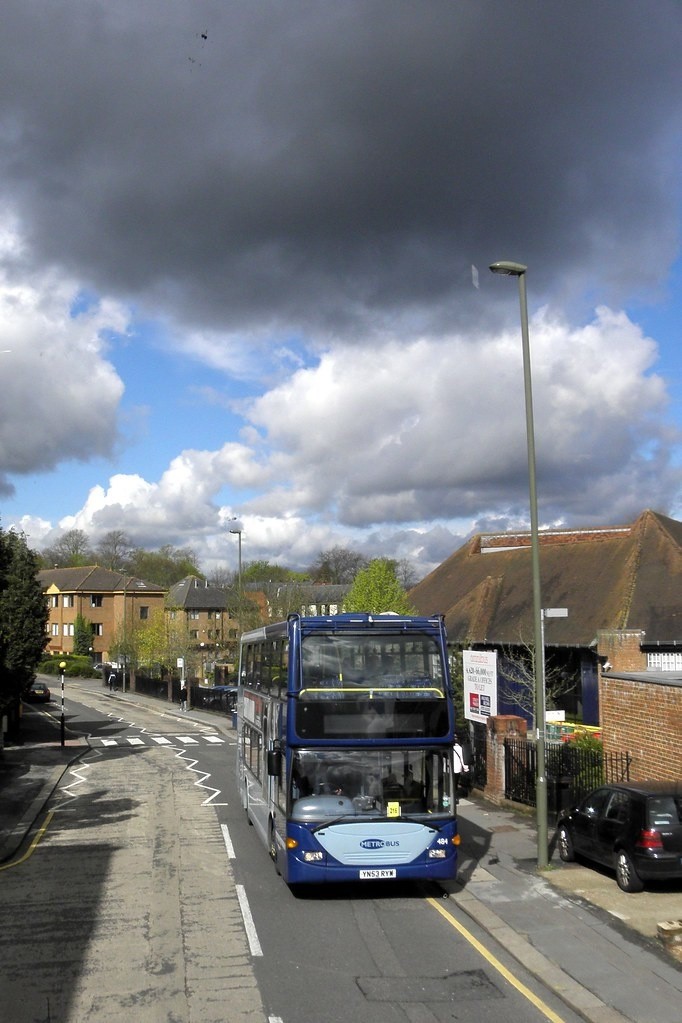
[230,529,244,636]
[488,260,547,868]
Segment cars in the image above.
[24,683,50,703]
[202,686,238,703]
[93,661,121,670]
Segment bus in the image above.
[236,613,474,891]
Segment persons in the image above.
[442,734,464,801]
[108,672,116,691]
[343,657,353,672]
[366,656,379,678]
[381,656,401,677]
[179,686,187,712]
[309,761,344,795]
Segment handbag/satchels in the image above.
[458,772,470,786]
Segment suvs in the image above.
[557,780,682,893]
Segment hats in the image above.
[404,764,413,775]
